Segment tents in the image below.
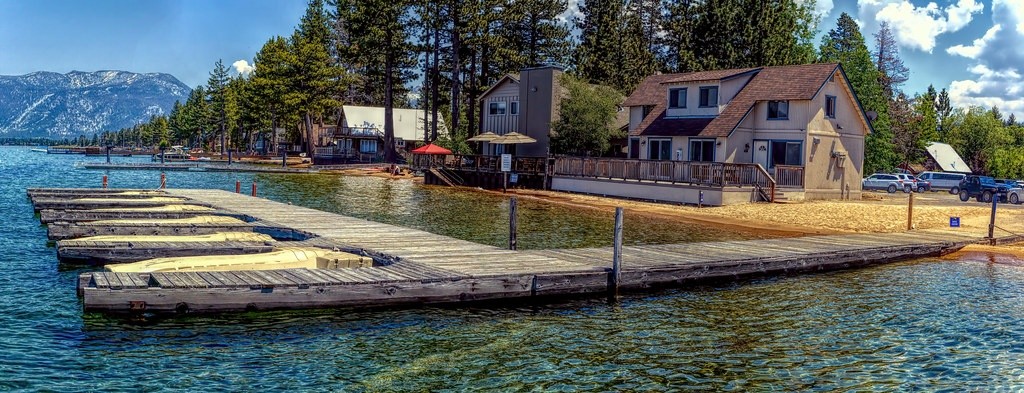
[411,144,451,155]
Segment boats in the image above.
[31,149,46,153]
[156,145,198,161]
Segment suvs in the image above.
[958,175,1008,203]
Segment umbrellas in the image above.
[467,131,500,155]
[489,132,537,155]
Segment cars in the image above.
[863,174,904,193]
[891,169,931,193]
[994,179,1024,204]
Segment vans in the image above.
[915,172,966,195]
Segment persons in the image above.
[391,165,400,176]
